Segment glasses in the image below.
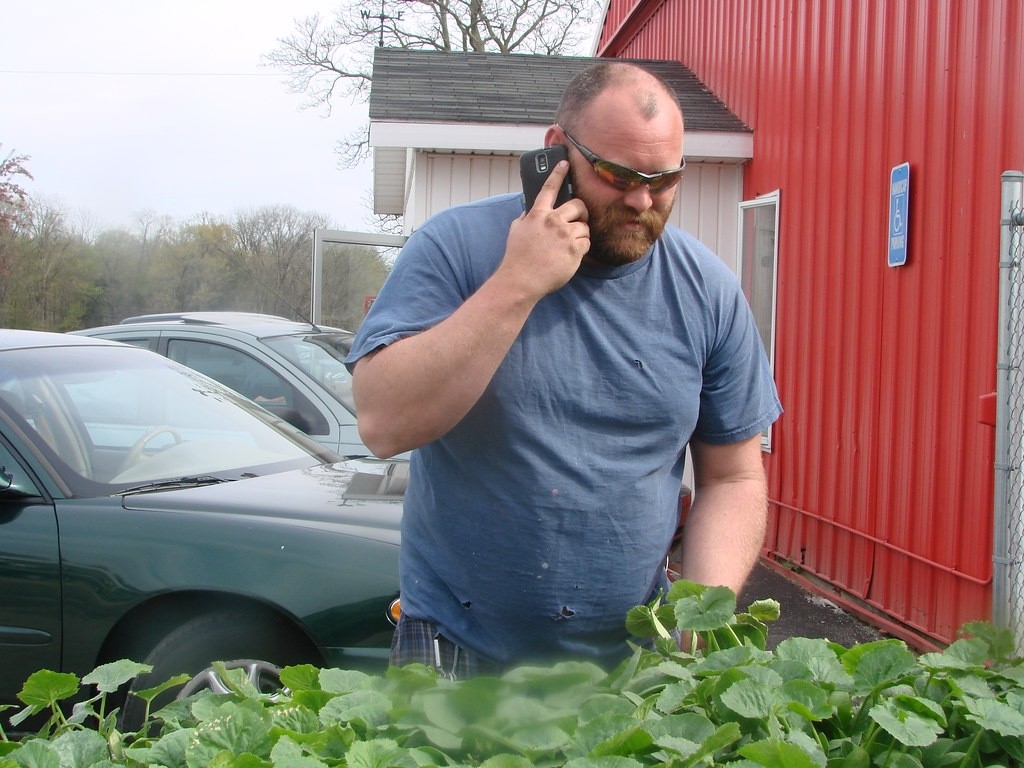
[556,123,685,194]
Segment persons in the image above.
[343,65,786,679]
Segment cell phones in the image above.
[519,143,575,216]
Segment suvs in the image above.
[0,313,413,463]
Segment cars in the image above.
[0,329,410,748]
